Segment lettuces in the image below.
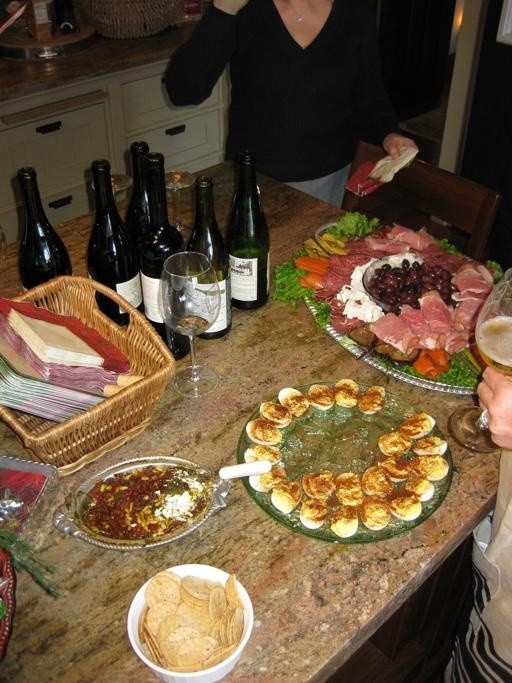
[273,211,506,392]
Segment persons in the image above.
[161,0,418,212]
[439,362,511,682]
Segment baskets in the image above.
[88,0,176,40]
[0,277,175,477]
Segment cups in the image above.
[127,562,256,683]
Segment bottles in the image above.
[120,141,147,218]
[82,160,134,309]
[8,162,69,281]
[229,155,273,307]
[191,175,230,338]
[134,155,185,357]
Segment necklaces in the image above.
[285,0,317,24]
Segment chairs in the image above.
[342,141,501,261]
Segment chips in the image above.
[134,568,244,674]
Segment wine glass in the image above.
[161,251,223,398]
[450,270,512,454]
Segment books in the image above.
[0,296,146,423]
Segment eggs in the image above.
[243,378,451,539]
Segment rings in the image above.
[473,408,491,430]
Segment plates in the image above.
[50,455,234,553]
[237,383,454,544]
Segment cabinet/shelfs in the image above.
[0,84,120,245]
[117,54,228,173]
